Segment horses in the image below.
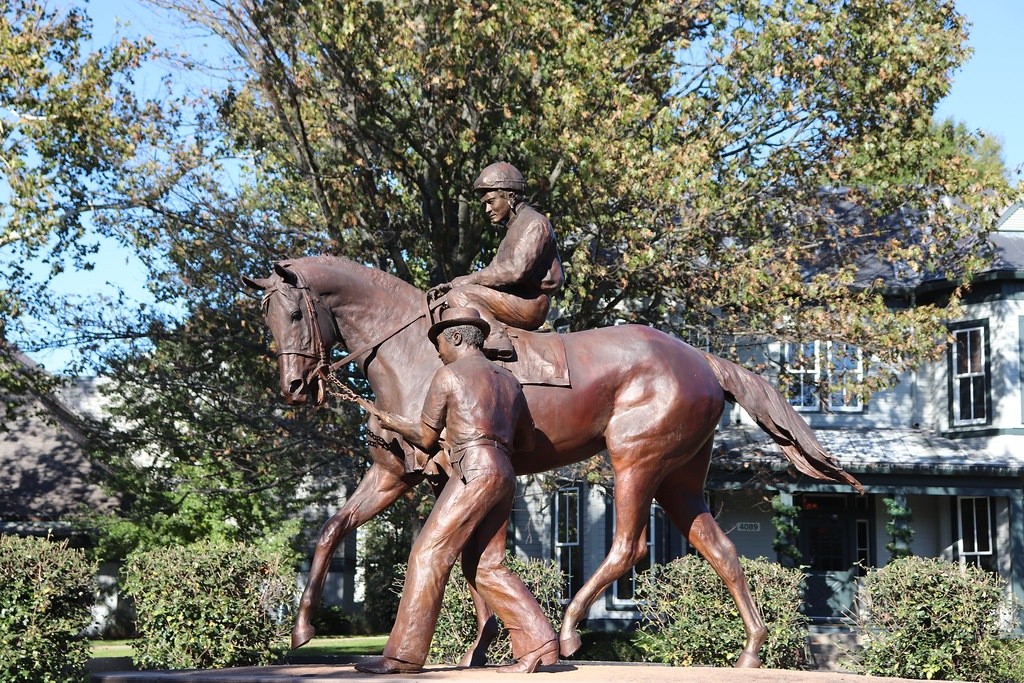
[241,253,866,670]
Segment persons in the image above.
[445,162,564,361]
[355,307,560,673]
